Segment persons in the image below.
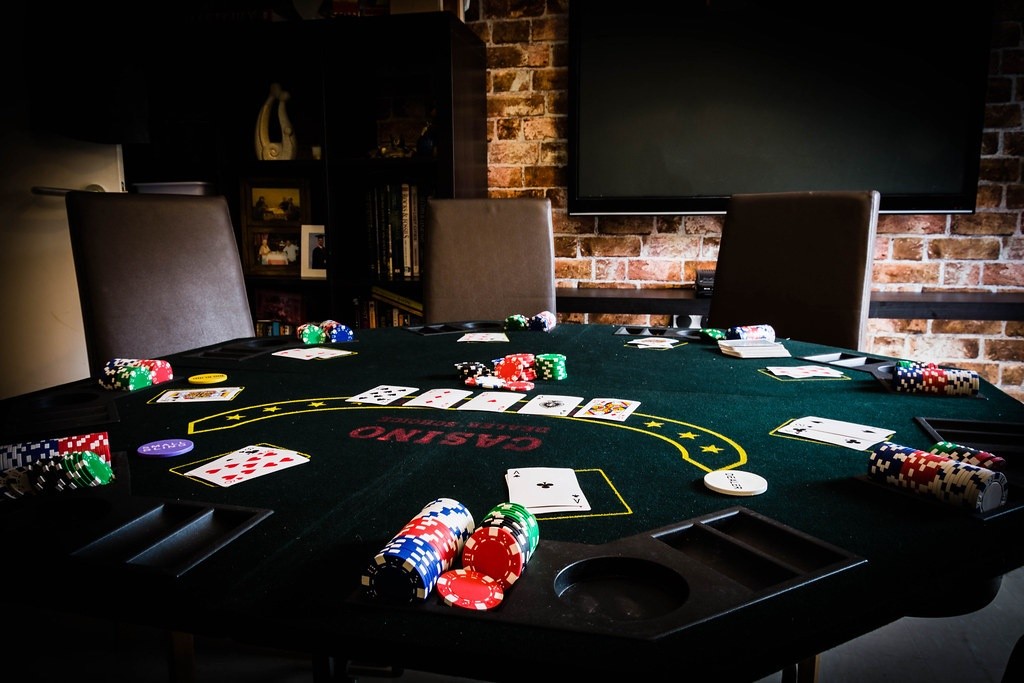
[257,237,299,267]
[256,194,299,223]
[312,234,326,269]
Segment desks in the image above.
[556,288,1024,323]
[0,325,1024,683]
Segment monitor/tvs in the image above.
[566,0,995,217]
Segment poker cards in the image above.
[765,364,846,379]
[717,338,792,359]
[345,384,643,423]
[777,415,897,452]
[504,465,592,515]
[456,332,511,343]
[155,387,241,403]
[626,336,679,350]
[271,347,355,361]
[183,444,311,488]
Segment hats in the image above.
[314,234,325,238]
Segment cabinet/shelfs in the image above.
[212,46,336,325]
[341,155,429,326]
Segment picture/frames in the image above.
[247,185,305,225]
[248,228,301,270]
[300,225,326,280]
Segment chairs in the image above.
[64,190,256,377]
[418,197,556,321]
[706,190,880,347]
